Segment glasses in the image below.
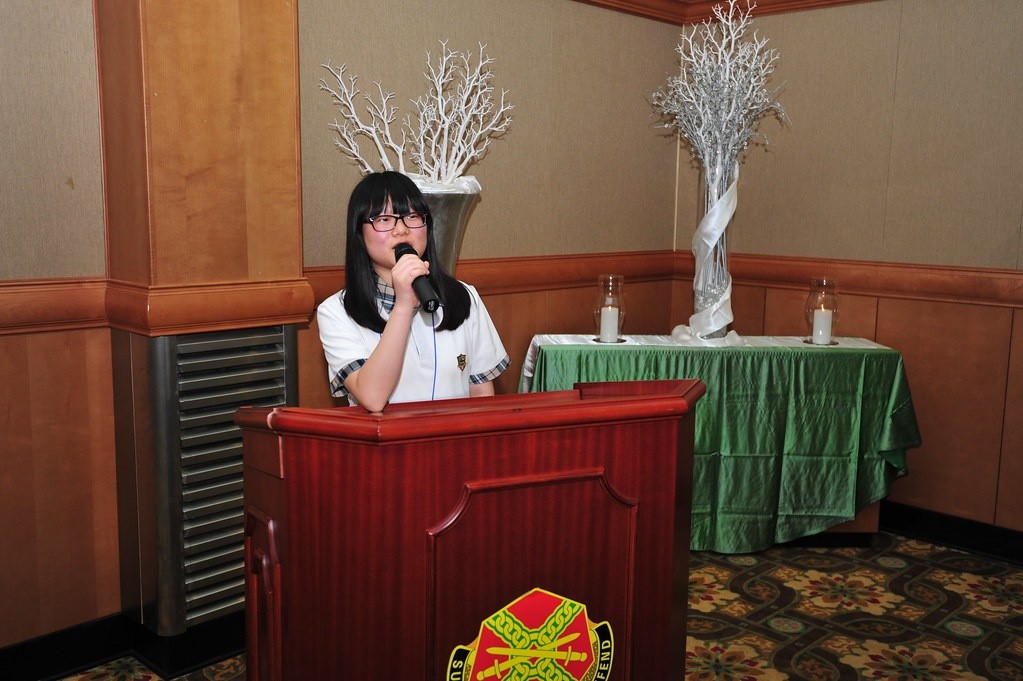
[363,211,429,231]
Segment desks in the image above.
[517,333,922,555]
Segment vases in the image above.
[693,158,740,339]
[421,193,476,276]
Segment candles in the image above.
[599,305,619,342]
[811,304,833,345]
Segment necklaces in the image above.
[380,311,437,401]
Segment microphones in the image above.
[395,243,439,313]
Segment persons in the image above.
[317,170,511,412]
